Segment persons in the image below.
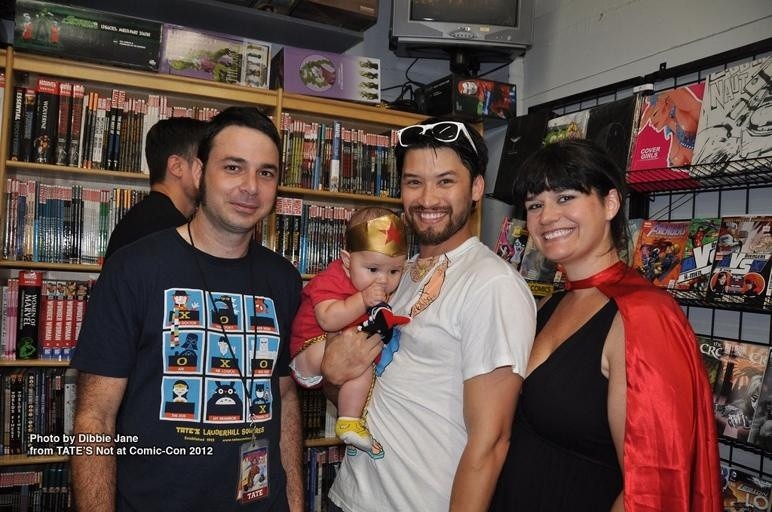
[326,116,537,512]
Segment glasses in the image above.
[397,121,478,156]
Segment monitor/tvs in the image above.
[389,0,533,62]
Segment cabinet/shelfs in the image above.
[494,31,771,512]
[0,42,485,463]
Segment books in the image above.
[495,54,772,512]
[0,62,418,512]
